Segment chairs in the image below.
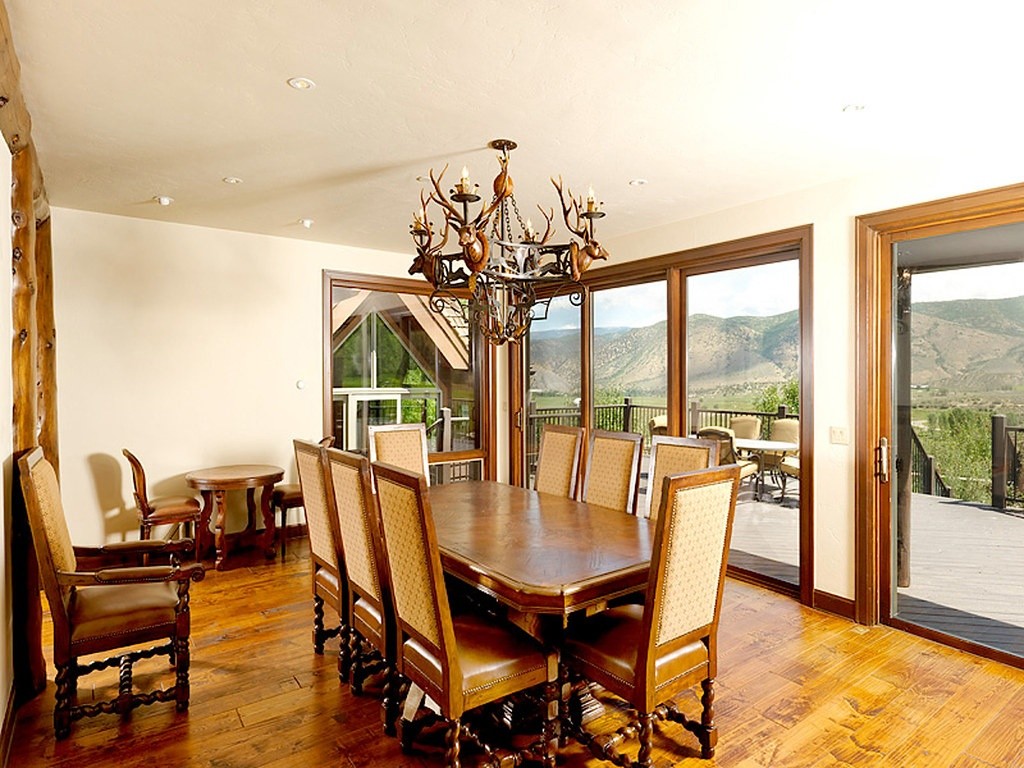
[16,444,210,739]
[293,413,802,766]
[273,434,336,566]
[123,448,203,563]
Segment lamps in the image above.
[408,140,610,348]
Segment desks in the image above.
[689,432,798,506]
[185,465,285,577]
[426,478,659,768]
